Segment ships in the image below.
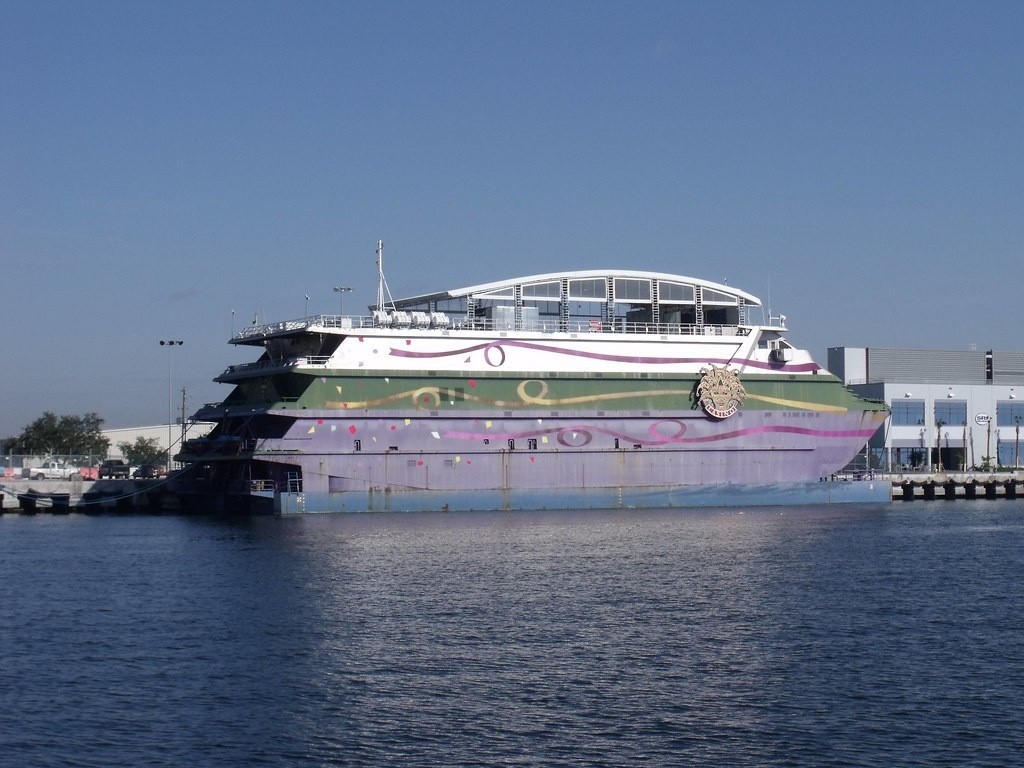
[171,240,896,511]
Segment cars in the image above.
[133,465,160,479]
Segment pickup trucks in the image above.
[22,461,78,481]
[98,460,129,480]
